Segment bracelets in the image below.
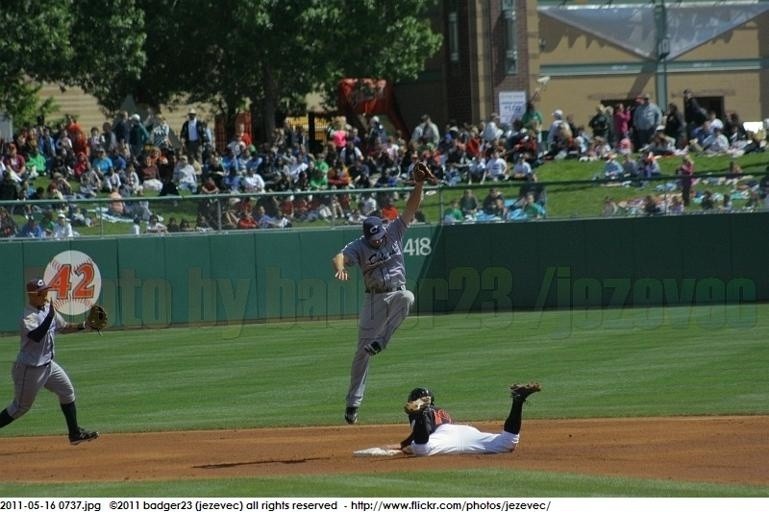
[77,321,86,331]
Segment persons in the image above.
[399,382,541,457]
[0,278,99,446]
[332,171,427,425]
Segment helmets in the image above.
[408,387,434,403]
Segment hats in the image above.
[25,279,51,294]
[363,216,388,240]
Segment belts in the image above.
[364,288,404,294]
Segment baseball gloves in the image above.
[413,161,438,185]
[85,305,109,334]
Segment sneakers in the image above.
[345,406,359,424]
[511,382,541,399]
[69,428,99,445]
[364,341,381,356]
[405,396,431,416]
